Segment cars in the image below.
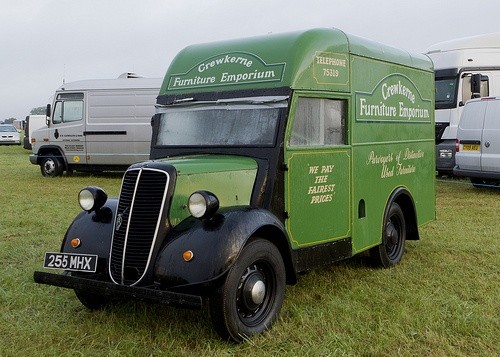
[0,123,23,145]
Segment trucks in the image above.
[422,34,500,191]
[29,29,435,341]
